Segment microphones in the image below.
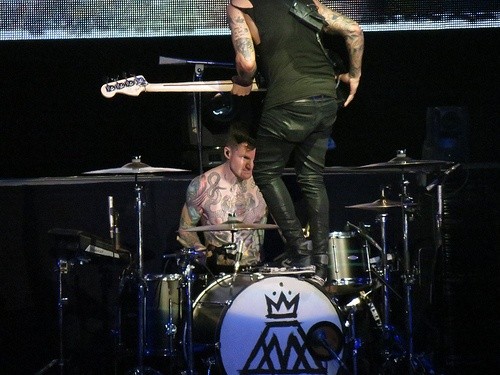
[426,163,460,191]
[312,329,350,371]
[208,244,242,261]
[105,188,118,239]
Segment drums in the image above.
[179,273,348,375]
[120,272,225,358]
[326,230,374,286]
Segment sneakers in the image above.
[259,256,316,276]
[295,261,330,286]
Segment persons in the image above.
[226,0,364,287]
[178,120,269,276]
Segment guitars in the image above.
[99,72,346,106]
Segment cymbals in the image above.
[182,222,279,232]
[355,156,456,166]
[345,197,419,213]
[82,162,191,174]
